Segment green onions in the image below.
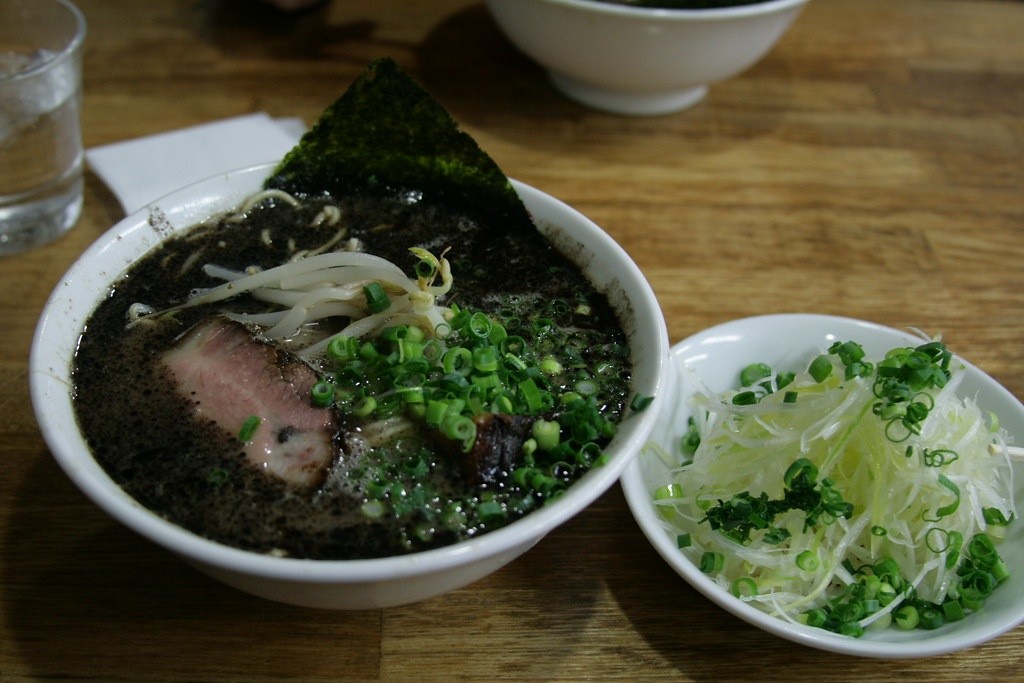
[655,341,1014,639]
[209,259,654,542]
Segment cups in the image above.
[0,0,88,257]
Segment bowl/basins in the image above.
[29,163,670,611]
[491,1,814,119]
[617,312,1024,657]
